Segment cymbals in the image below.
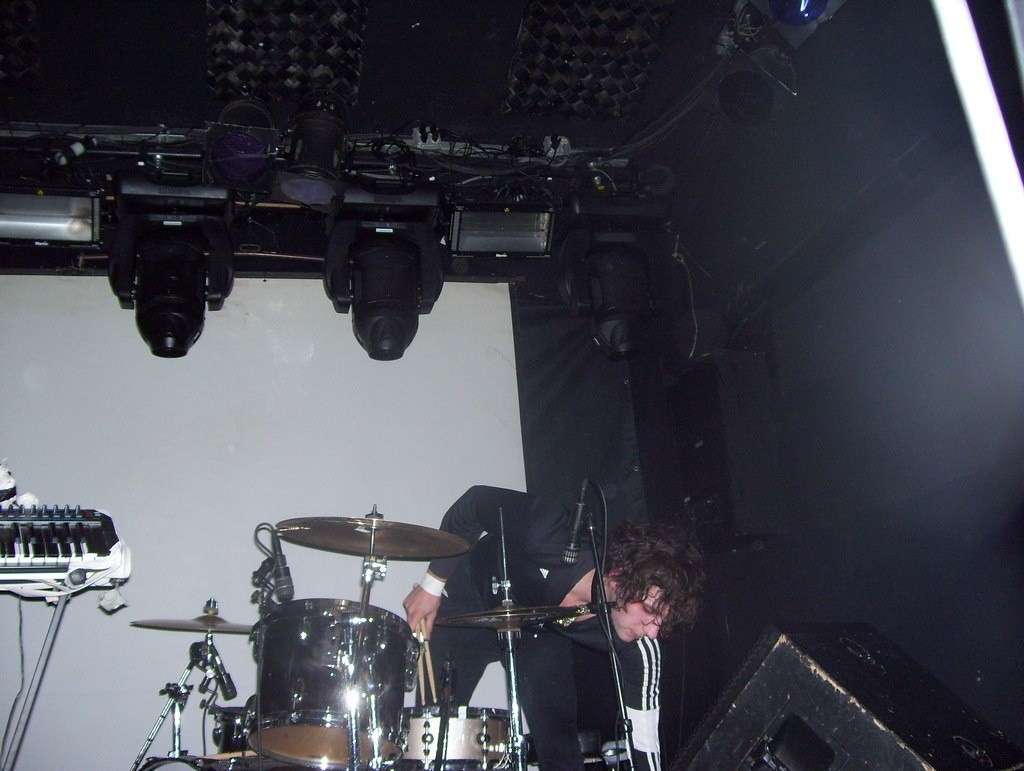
[276,516,474,560]
[127,615,255,636]
[433,602,581,628]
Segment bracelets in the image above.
[420,572,448,598]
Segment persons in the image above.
[402,484,706,771]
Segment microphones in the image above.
[560,478,588,565]
[212,646,237,701]
[270,530,294,603]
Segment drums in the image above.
[397,706,512,770]
[138,754,235,771]
[251,597,421,771]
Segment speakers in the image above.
[669,623,1024,771]
